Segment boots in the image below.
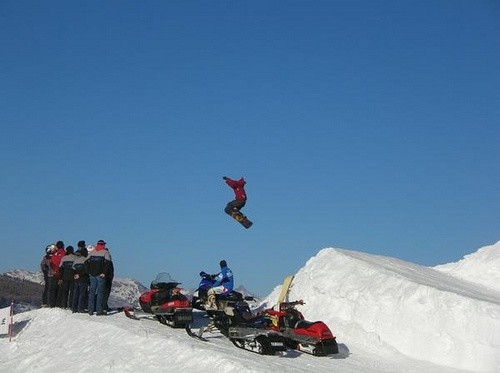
[206,294,217,309]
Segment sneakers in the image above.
[96,312,106,316]
[89,312,93,314]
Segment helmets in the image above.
[46,243,57,254]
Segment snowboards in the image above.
[224,204,253,229]
[278,275,294,302]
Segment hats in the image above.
[86,245,95,251]
[97,239,106,244]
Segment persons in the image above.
[75,240,88,256]
[41,245,52,303]
[207,259,234,309]
[50,240,66,307]
[223,175,247,211]
[59,245,77,307]
[71,249,88,313]
[85,240,113,315]
